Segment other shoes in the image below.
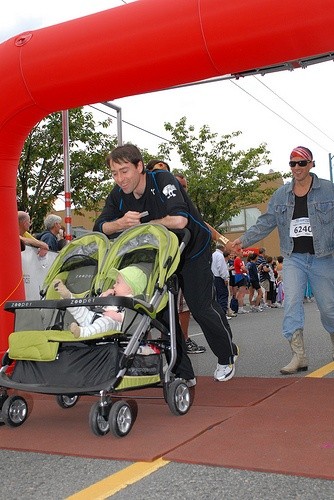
[253,306,263,312]
[273,303,284,308]
[249,304,263,309]
[228,313,237,317]
[238,309,249,313]
[226,316,232,319]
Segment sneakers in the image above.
[185,338,206,354]
[214,344,239,381]
[173,377,196,387]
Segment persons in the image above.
[36,214,65,251]
[225,248,284,315]
[53,266,147,339]
[303,276,316,303]
[233,146,334,373]
[17,211,49,257]
[171,174,241,354]
[146,159,171,172]
[211,243,230,319]
[93,143,240,382]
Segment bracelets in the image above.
[217,236,230,246]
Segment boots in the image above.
[280,328,308,374]
[330,332,334,359]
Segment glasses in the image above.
[289,160,311,167]
[182,185,187,188]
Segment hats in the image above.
[147,160,170,172]
[108,265,148,296]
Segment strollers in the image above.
[0,221,192,438]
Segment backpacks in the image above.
[230,295,239,314]
